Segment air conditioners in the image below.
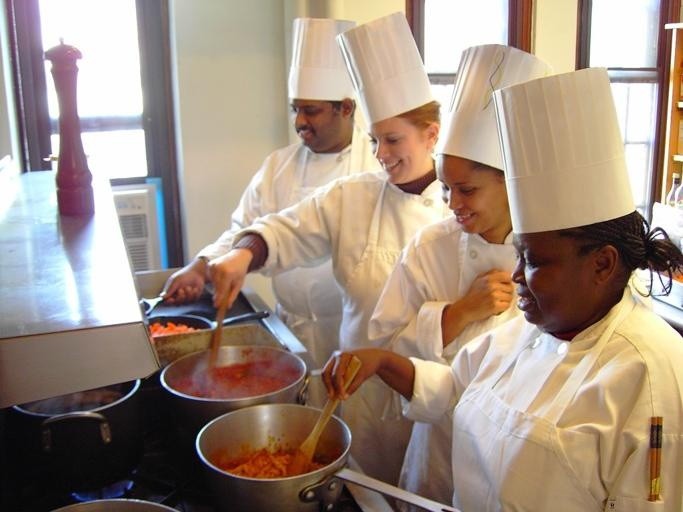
[114,190,153,272]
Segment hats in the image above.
[335,12,434,124]
[435,43,550,172]
[492,67,637,235]
[287,19,356,103]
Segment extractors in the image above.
[1,155,163,411]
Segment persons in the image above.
[320,208,682,512]
[157,97,386,416]
[364,151,527,511]
[203,100,455,504]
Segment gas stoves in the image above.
[0,287,363,511]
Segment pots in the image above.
[7,378,200,511]
[195,404,464,512]
[158,343,313,423]
[148,309,272,365]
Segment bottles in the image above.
[665,172,683,209]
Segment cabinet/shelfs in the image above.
[660,21,682,205]
